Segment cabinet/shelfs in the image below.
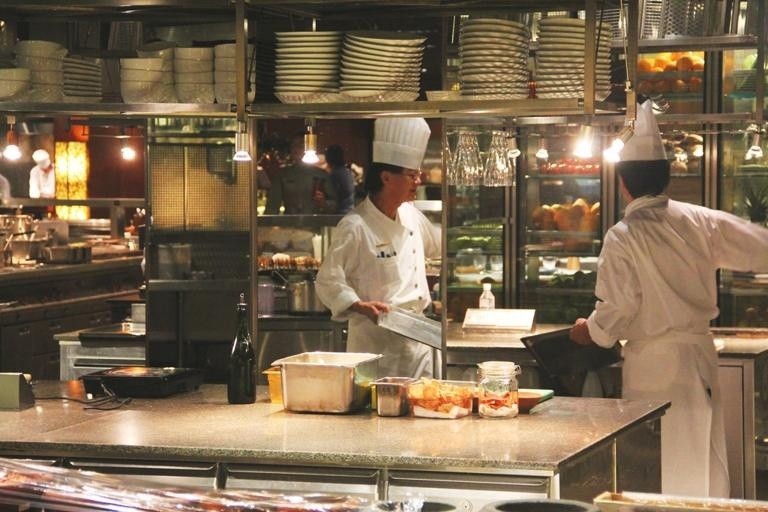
[242,15,446,111]
[446,15,767,332]
[144,128,252,383]
[446,362,747,504]
[9,16,241,110]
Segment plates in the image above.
[518,388,555,412]
[458,18,531,100]
[62,55,104,103]
[520,326,623,381]
[377,307,443,351]
[274,30,342,104]
[339,29,428,101]
[77,322,146,337]
[534,17,613,102]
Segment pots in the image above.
[285,280,330,314]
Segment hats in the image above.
[372,115,431,171]
[618,98,668,162]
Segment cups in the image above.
[483,129,515,186]
[447,129,483,186]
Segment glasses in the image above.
[405,171,423,179]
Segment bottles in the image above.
[110,201,127,237]
[227,304,256,404]
[480,283,494,310]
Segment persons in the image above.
[321,124,358,214]
[27,150,55,199]
[315,118,442,379]
[262,136,334,215]
[569,99,768,501]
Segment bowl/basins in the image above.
[174,46,214,61]
[214,83,256,104]
[173,59,214,72]
[175,72,213,84]
[215,72,256,83]
[1,68,30,80]
[214,57,256,71]
[121,81,163,103]
[175,84,214,102]
[16,38,69,59]
[135,40,174,101]
[270,351,385,414]
[15,56,62,71]
[119,58,164,71]
[214,42,256,58]
[32,69,62,84]
[24,84,63,103]
[121,70,163,82]
[1,82,28,99]
